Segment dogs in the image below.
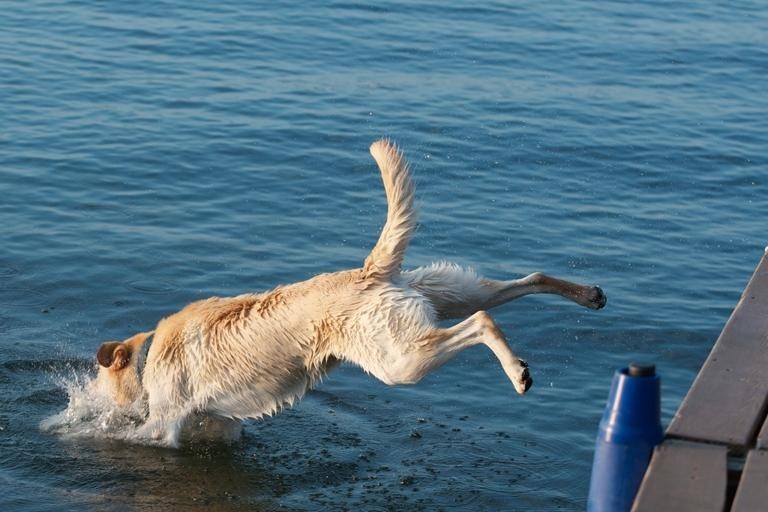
[87,138,609,447]
